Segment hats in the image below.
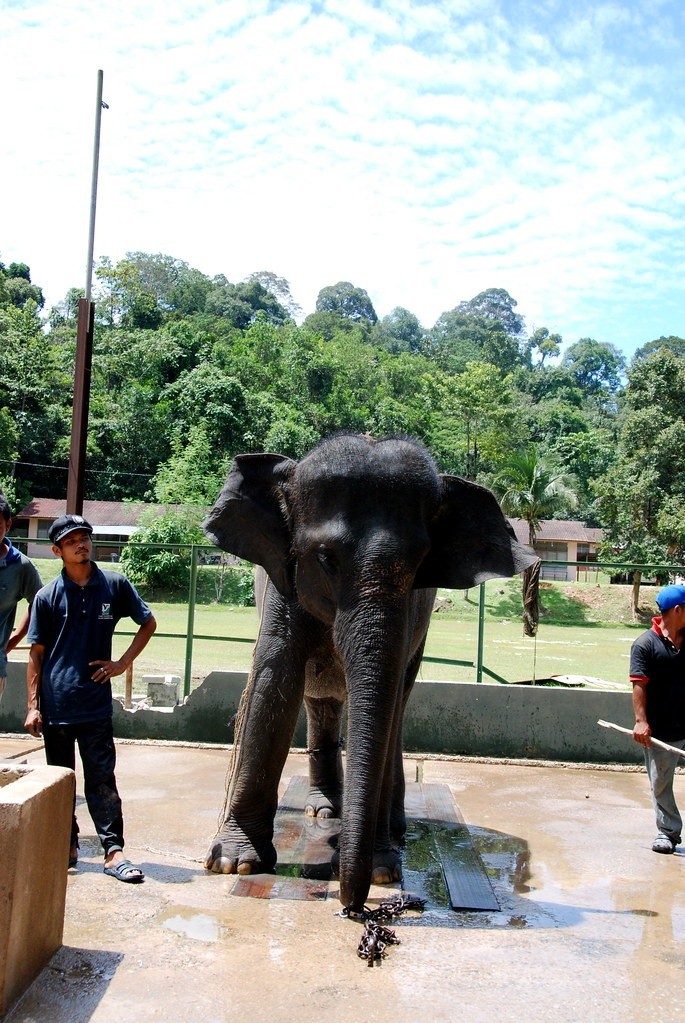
[48,514,93,544]
[655,585,685,611]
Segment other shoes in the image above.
[651,830,679,854]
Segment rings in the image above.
[100,667,104,672]
[104,670,107,676]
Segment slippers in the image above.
[104,860,144,881]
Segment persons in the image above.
[629,585,684,852]
[22,515,156,882]
[0,501,43,699]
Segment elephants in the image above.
[202,436,541,906]
